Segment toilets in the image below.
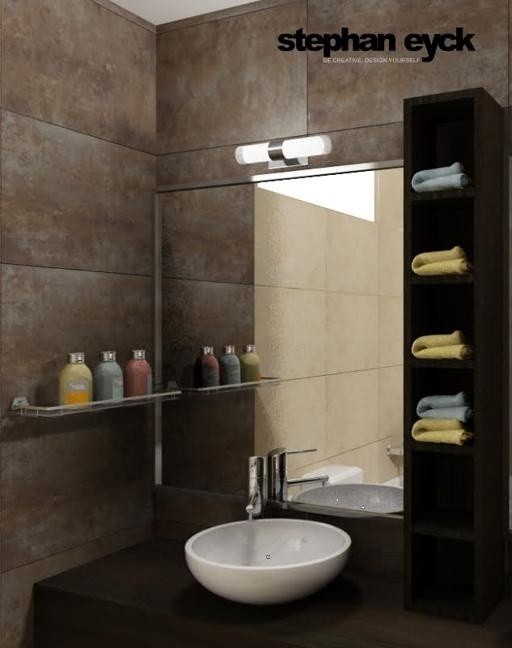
[301,463,363,490]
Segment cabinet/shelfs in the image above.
[401,87,504,618]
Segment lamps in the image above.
[234,134,331,171]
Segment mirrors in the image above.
[151,158,402,520]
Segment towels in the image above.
[410,245,473,276]
[407,159,468,193]
[411,385,474,448]
[409,324,471,363]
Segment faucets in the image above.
[246,456,264,519]
[267,449,328,502]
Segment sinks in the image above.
[184,518,352,606]
[296,483,404,513]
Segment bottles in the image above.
[123,349,153,405]
[219,345,241,392]
[92,350,124,408]
[193,345,220,394]
[57,351,93,413]
[238,344,262,389]
